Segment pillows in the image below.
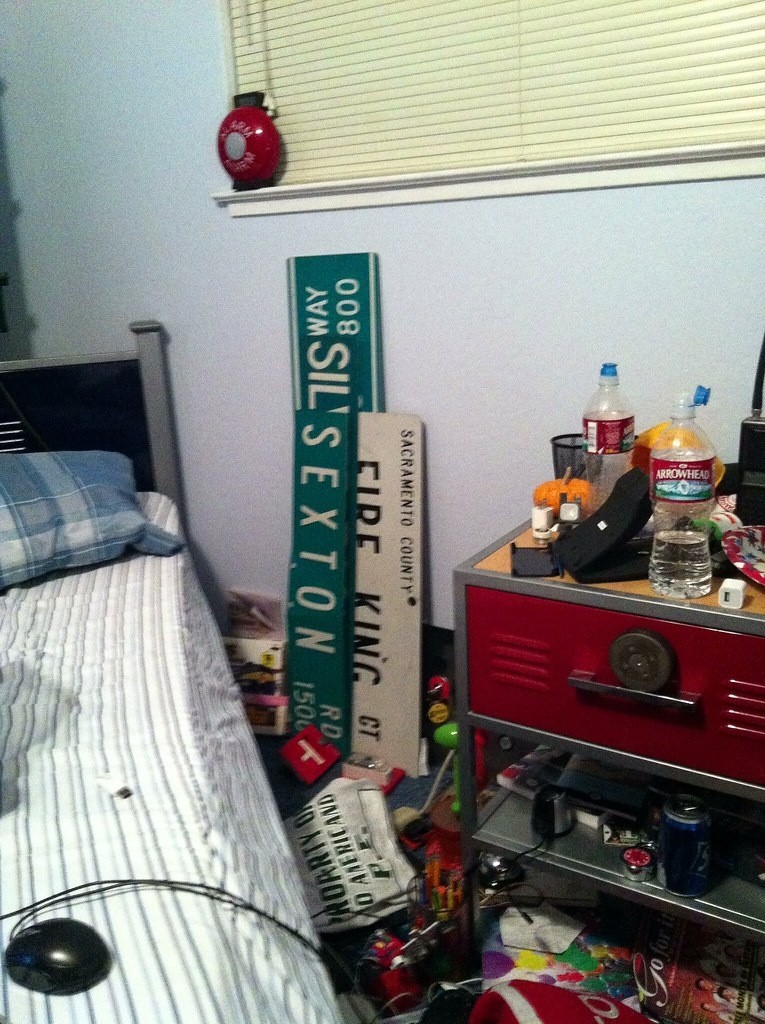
[0,449,186,591]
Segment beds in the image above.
[1,319,348,1023]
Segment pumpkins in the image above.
[533,467,594,518]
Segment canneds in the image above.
[657,794,710,898]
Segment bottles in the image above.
[583,361,636,513]
[648,399,713,598]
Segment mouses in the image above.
[5,917,112,996]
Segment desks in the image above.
[452,518,765,943]
